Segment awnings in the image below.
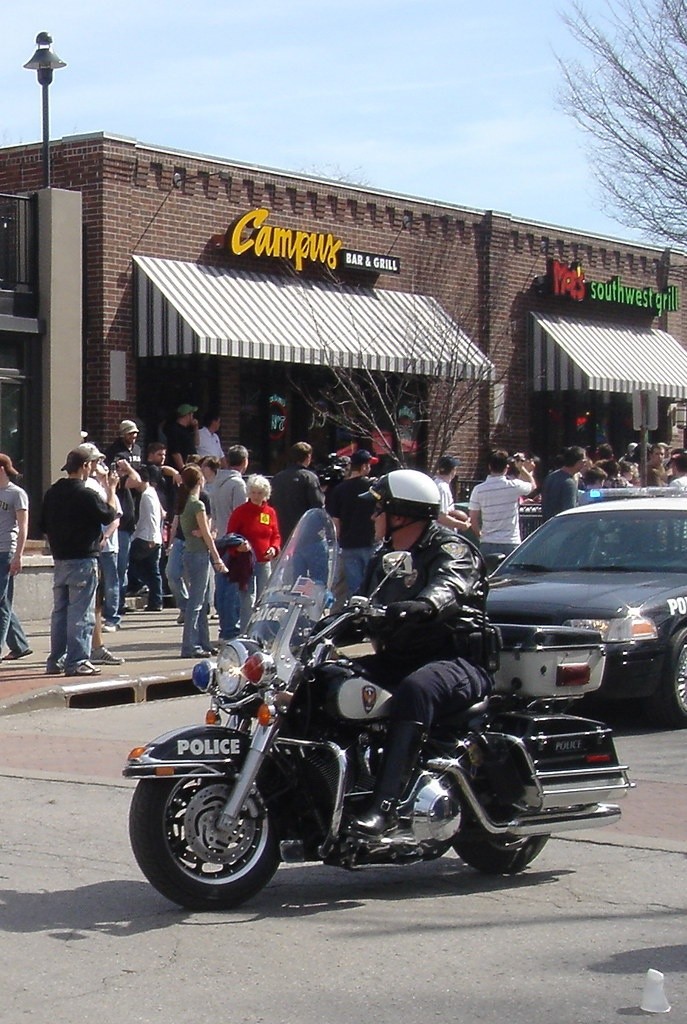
[132,253,497,382]
[530,310,687,400]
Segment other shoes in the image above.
[119,605,136,615]
[125,585,149,597]
[69,659,101,674]
[182,642,219,658]
[177,611,185,623]
[47,661,66,675]
[143,605,162,611]
[207,611,219,619]
[218,637,229,646]
[101,624,122,632]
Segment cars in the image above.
[486,488,687,728]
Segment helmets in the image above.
[357,469,442,520]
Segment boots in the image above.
[348,718,430,838]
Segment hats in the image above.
[0,454,19,476]
[439,456,462,471]
[351,450,379,466]
[177,404,199,416]
[61,446,92,471]
[118,420,140,435]
[79,441,107,462]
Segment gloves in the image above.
[384,600,436,635]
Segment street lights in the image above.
[23,30,69,189]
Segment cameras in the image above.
[223,567,230,573]
[510,454,519,462]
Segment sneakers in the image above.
[89,645,125,665]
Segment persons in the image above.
[549,444,635,506]
[314,469,496,840]
[617,442,687,498]
[542,447,586,524]
[328,450,471,593]
[432,456,471,535]
[469,446,537,576]
[42,403,281,677]
[271,441,330,589]
[506,452,542,505]
[0,453,33,663]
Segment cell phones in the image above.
[111,462,116,473]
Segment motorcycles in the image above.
[122,508,638,909]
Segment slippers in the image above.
[2,649,33,661]
